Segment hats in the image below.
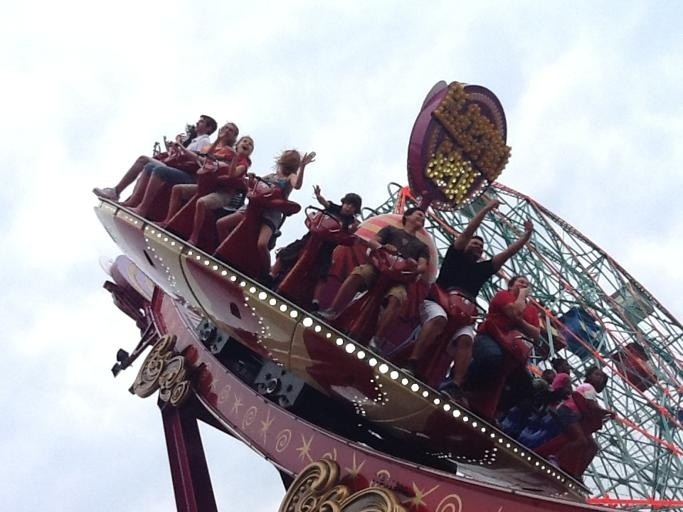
[548,373,570,392]
[341,194,361,214]
[577,383,597,401]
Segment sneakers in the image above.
[368,338,385,356]
[312,309,336,322]
[402,358,418,377]
[440,383,459,400]
[93,187,121,201]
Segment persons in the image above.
[92,112,618,483]
